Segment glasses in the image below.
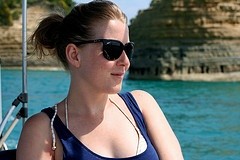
[71,39,135,62]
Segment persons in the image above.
[15,0,183,160]
